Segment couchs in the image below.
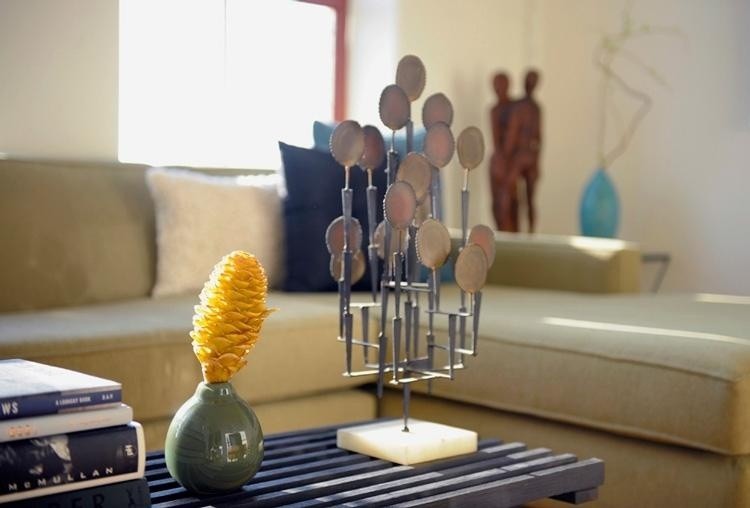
[2,151,385,457]
[379,226,749,508]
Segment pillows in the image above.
[314,117,455,288]
[140,165,284,300]
[280,127,409,293]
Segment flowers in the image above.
[191,251,278,383]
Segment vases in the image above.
[579,171,621,236]
[164,383,267,498]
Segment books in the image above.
[1,357,152,508]
